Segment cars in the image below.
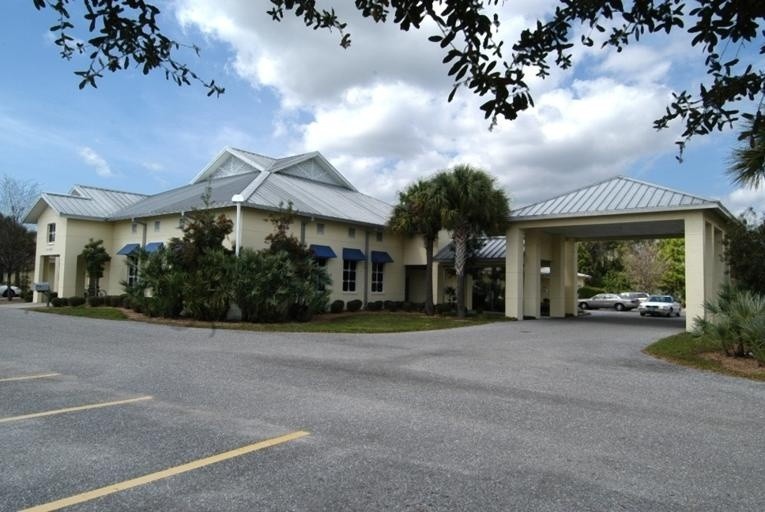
[620,291,649,302]
[639,295,681,319]
[0,284,21,297]
[577,293,640,312]
